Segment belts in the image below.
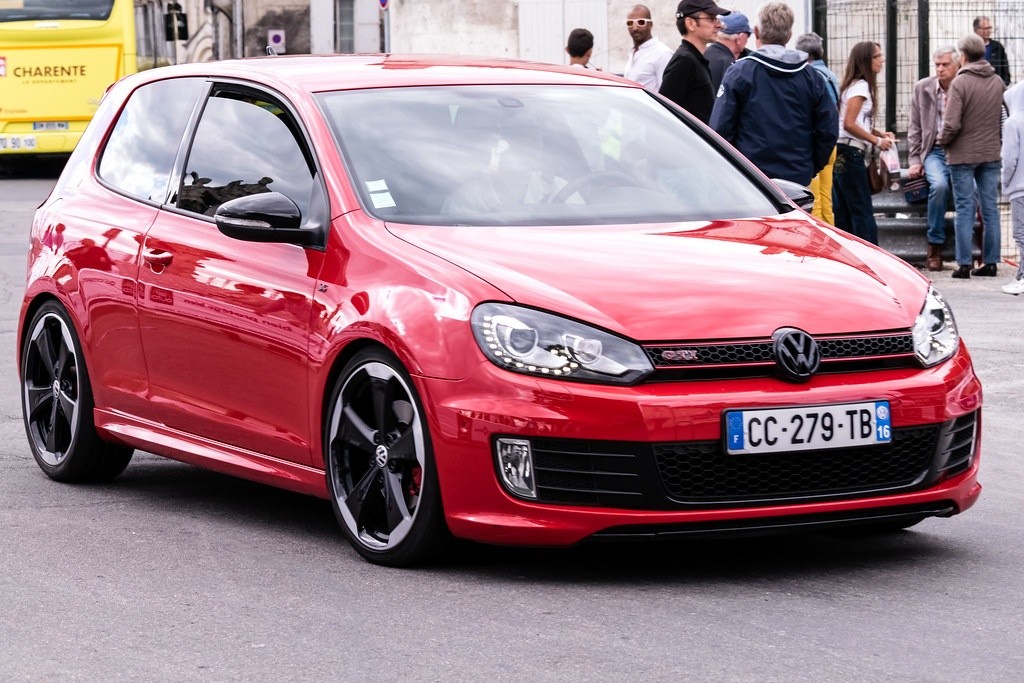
[835,137,866,151]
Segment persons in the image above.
[622,4,675,94]
[1001,80,1024,294]
[708,4,839,214]
[973,16,1010,87]
[796,32,840,227]
[703,11,755,93]
[832,41,895,246]
[936,34,1004,278]
[565,28,603,70]
[658,0,731,125]
[907,46,980,271]
[440,129,587,213]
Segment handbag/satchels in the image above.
[879,134,901,192]
[866,160,882,196]
[903,172,929,204]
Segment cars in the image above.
[15,54,982,566]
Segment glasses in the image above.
[977,26,992,31]
[743,32,751,38]
[873,52,884,61]
[685,16,720,23]
[626,18,653,27]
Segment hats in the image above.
[676,0,731,18]
[796,32,822,51]
[717,11,753,33]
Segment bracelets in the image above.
[876,137,881,147]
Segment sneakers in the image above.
[974,264,996,276]
[952,264,970,278]
[928,243,943,271]
[1002,277,1024,294]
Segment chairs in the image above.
[272,105,502,203]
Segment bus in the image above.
[0,0,188,177]
